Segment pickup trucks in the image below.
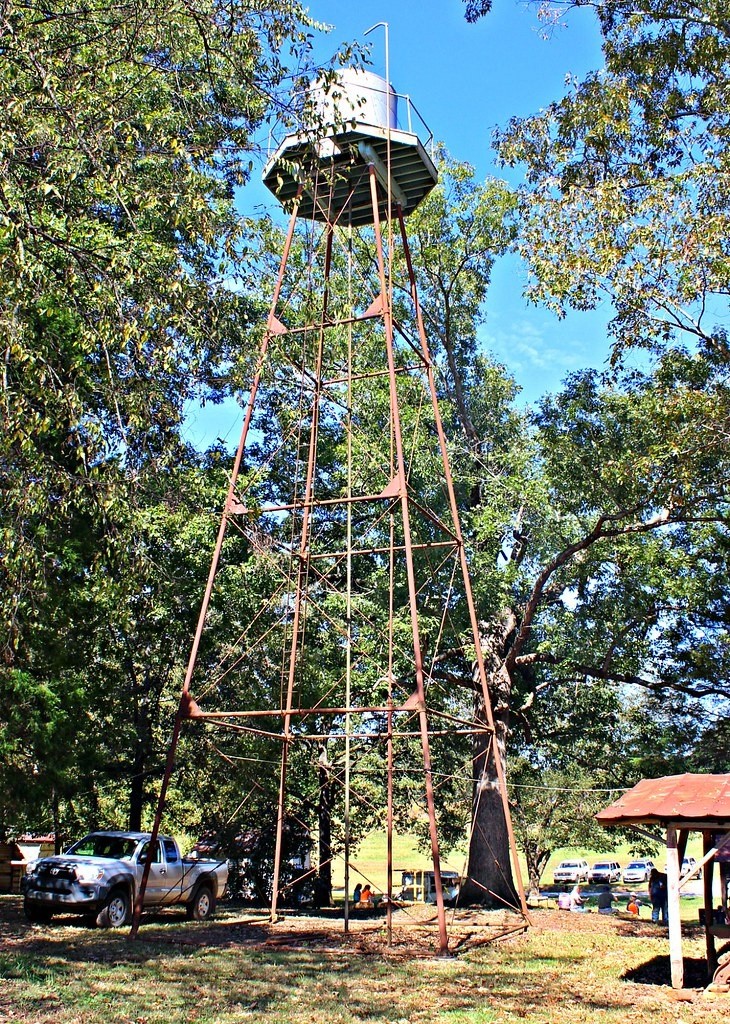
[19,830,228,929]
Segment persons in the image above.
[627,893,652,917]
[598,886,619,914]
[353,884,362,903]
[360,885,375,903]
[570,886,592,913]
[649,869,668,923]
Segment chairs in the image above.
[107,842,143,860]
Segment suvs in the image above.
[553,859,590,884]
[623,859,656,883]
[590,860,622,884]
[663,857,702,880]
